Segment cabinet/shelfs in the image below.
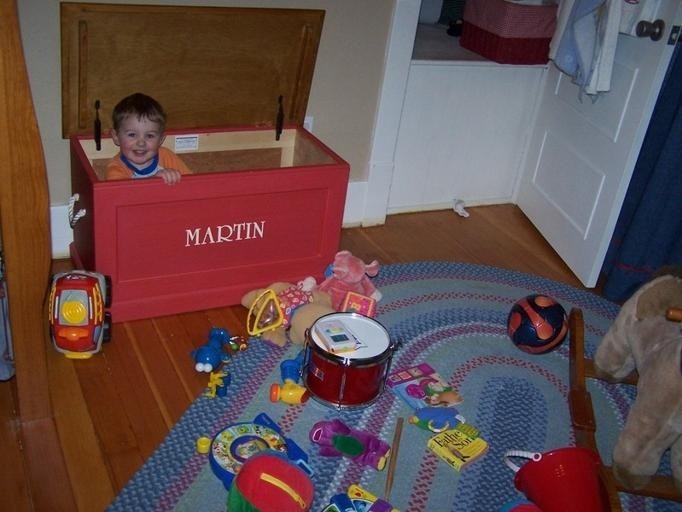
[303,0,573,217]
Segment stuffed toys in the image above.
[320,251,382,315]
[408,406,480,440]
[309,419,391,469]
[228,455,314,511]
[567,269,681,511]
[240,277,338,348]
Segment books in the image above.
[427,428,489,472]
[384,363,464,411]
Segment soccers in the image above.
[508,294,568,354]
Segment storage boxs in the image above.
[59,1,352,327]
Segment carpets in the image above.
[97,261,681,509]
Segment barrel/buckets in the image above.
[504,447,608,512]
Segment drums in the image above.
[302,312,397,411]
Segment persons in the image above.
[104,93,196,189]
